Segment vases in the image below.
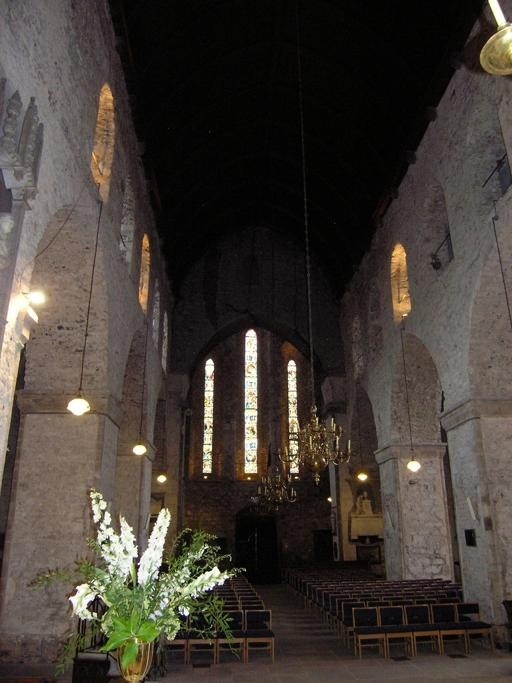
[116,637,156,681]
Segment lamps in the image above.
[252,256,344,517]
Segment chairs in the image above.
[283,561,493,661]
[162,568,277,668]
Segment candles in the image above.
[487,0,506,27]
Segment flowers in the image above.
[31,484,249,678]
